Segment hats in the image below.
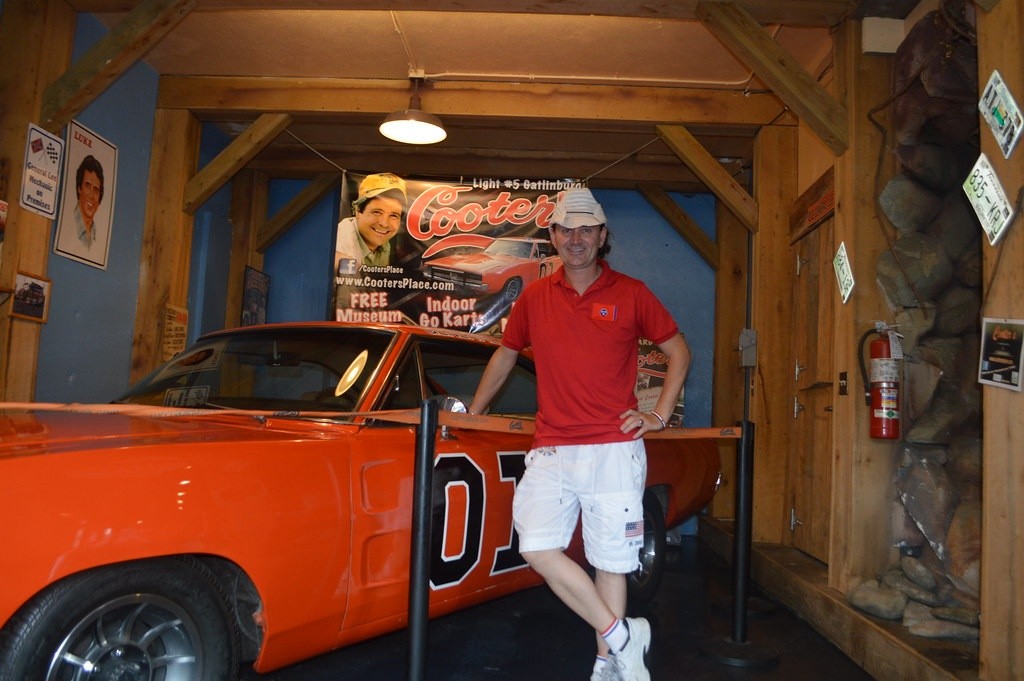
[352,173,408,210]
[549,187,607,231]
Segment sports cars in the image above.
[0,322,719,681]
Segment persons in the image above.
[334,173,407,322]
[468,189,689,681]
[73,155,104,251]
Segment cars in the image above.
[420,236,570,308]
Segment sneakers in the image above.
[608,617,653,681]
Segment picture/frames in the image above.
[8,267,53,324]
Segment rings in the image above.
[637,418,643,426]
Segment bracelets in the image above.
[651,410,666,428]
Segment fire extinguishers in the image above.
[857,318,905,440]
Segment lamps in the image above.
[378,68,448,145]
